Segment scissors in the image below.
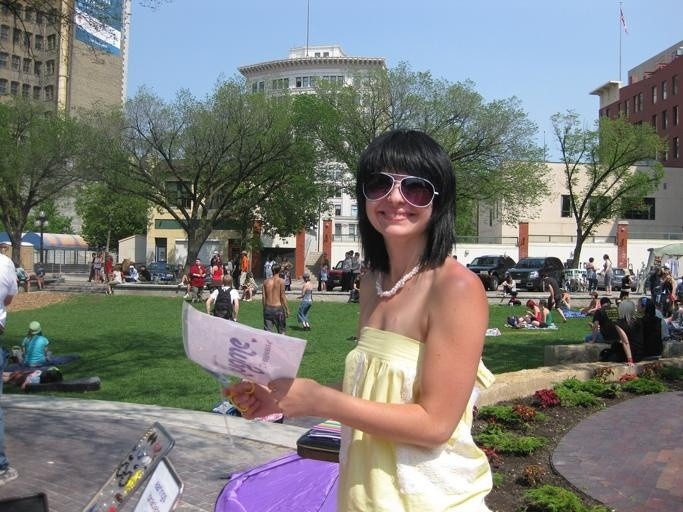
[204,369,254,412]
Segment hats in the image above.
[600,297,614,305]
[589,292,598,297]
[29,321,42,335]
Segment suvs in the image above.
[594,267,636,292]
[464,255,516,291]
[504,256,564,292]
[328,259,367,291]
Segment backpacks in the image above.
[213,286,233,319]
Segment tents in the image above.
[642,244,683,280]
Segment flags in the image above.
[619,8,629,35]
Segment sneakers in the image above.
[0,468,19,486]
[303,325,311,331]
[184,293,189,299]
[178,282,184,287]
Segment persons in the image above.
[13,261,46,292]
[219,127,499,511]
[175,250,314,335]
[1,241,20,487]
[320,250,361,291]
[453,253,683,369]
[88,250,151,296]
[17,320,49,367]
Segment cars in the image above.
[143,262,174,282]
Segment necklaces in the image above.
[375,262,428,298]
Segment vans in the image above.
[561,268,590,290]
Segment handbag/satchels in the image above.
[40,371,63,383]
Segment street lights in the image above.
[34,210,49,264]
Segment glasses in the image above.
[362,171,439,209]
